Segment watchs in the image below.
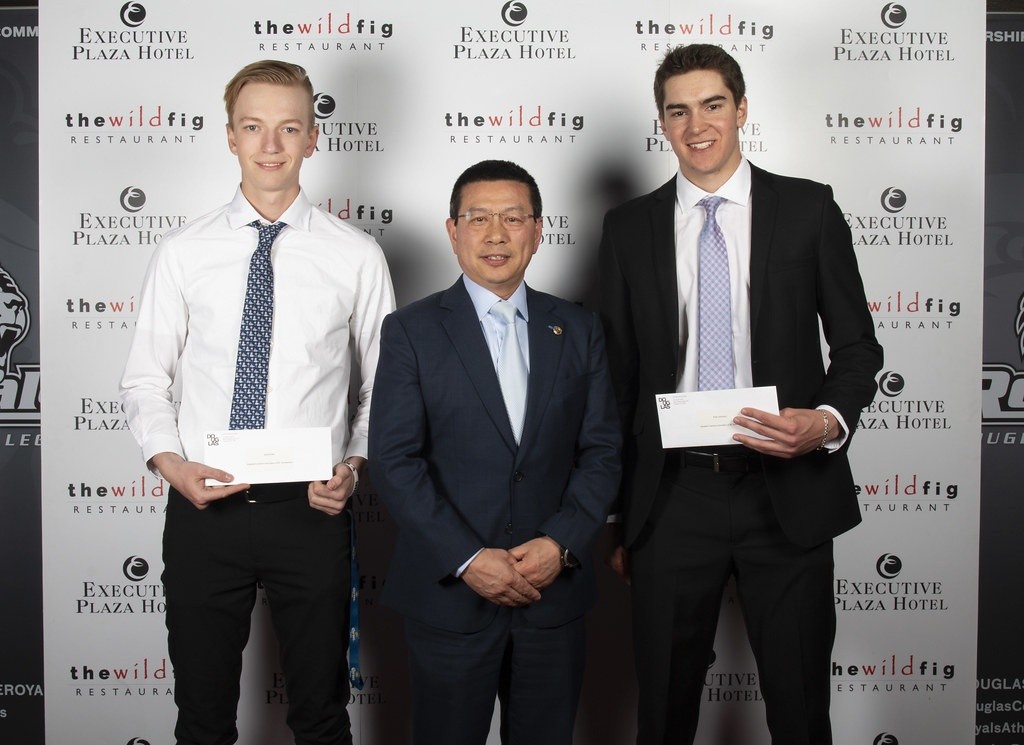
[559,545,581,570]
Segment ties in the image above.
[229,220,287,430]
[695,196,734,391]
[489,302,529,445]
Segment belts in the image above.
[667,448,777,472]
[227,483,307,505]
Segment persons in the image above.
[116,58,399,745]
[365,159,625,745]
[594,41,886,745]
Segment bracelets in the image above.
[817,408,829,451]
[344,461,360,499]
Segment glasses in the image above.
[457,213,535,231]
